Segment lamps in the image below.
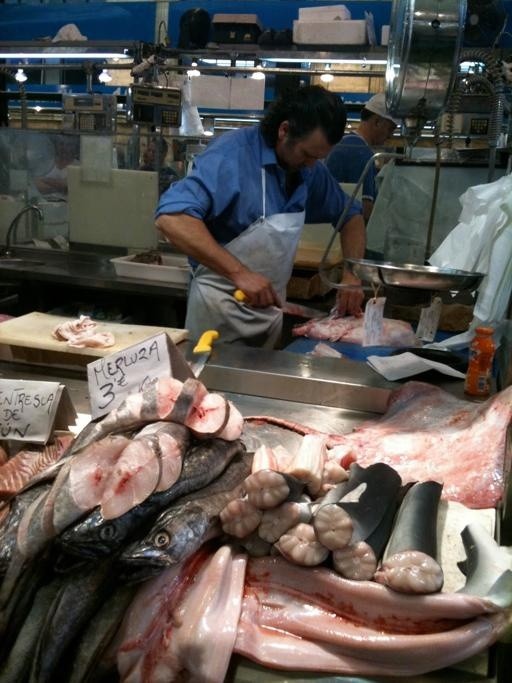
[14,61,28,82]
[320,64,334,83]
[99,60,112,83]
[251,62,266,80]
[186,58,200,76]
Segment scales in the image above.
[345,256,488,322]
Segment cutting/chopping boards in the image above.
[0,311,189,363]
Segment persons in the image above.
[37,139,80,202]
[325,91,399,226]
[154,80,366,350]
[134,140,168,177]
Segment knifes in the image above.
[186,329,220,381]
[237,290,329,321]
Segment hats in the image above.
[365,92,403,126]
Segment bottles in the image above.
[464,326,496,396]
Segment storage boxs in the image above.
[292,20,369,45]
[298,4,351,20]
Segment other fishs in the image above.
[0,373,512,683]
